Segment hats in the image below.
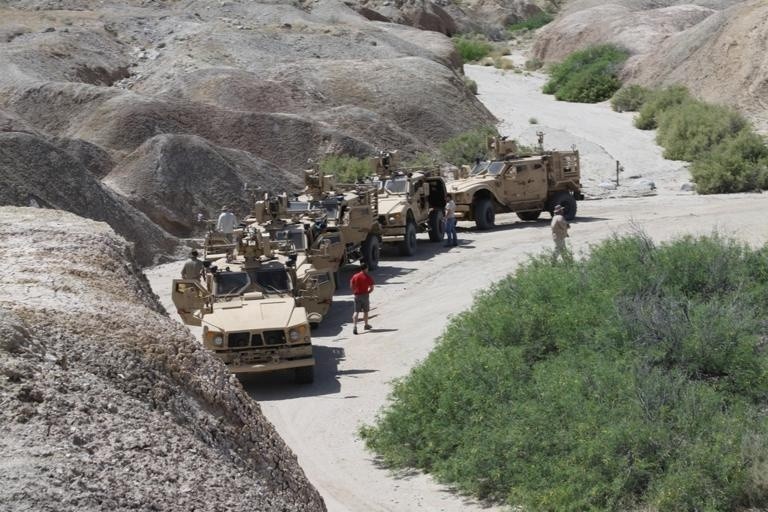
[553,205,564,212]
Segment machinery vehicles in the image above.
[204,190,345,294]
[444,129,581,230]
[282,155,380,272]
[166,228,335,387]
[353,143,449,255]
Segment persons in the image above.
[349,264,375,335]
[442,193,459,247]
[502,147,517,161]
[269,195,279,220]
[215,205,238,246]
[181,251,206,285]
[549,204,572,261]
